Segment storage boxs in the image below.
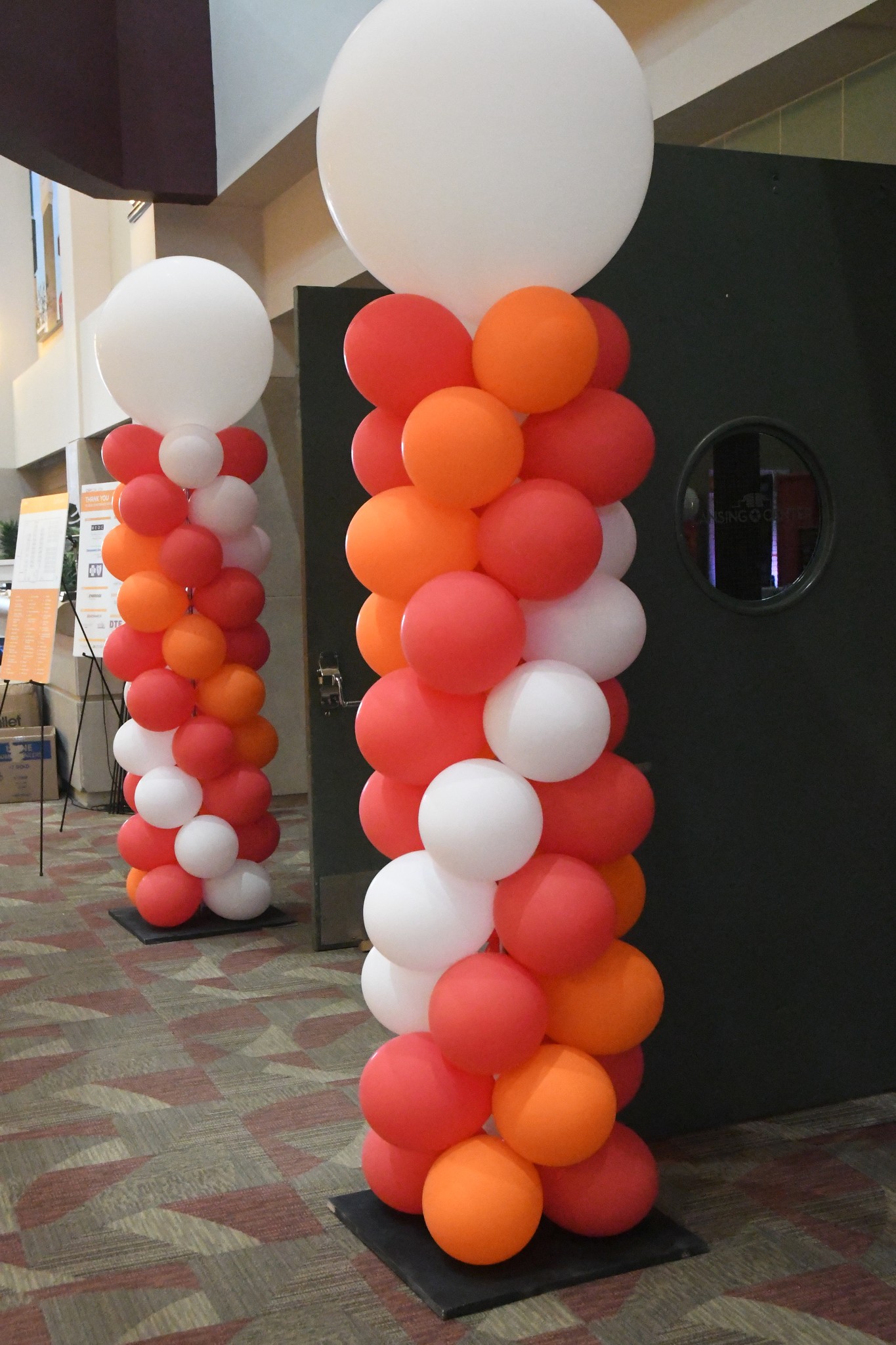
[0,663,59,805]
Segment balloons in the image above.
[316,0,657,332]
[343,294,478,416]
[571,296,630,393]
[94,255,274,436]
[101,424,167,486]
[101,476,284,868]
[136,867,204,929]
[126,867,147,909]
[472,287,599,415]
[215,427,268,484]
[201,859,272,922]
[401,385,523,511]
[158,424,224,489]
[352,408,416,496]
[344,478,658,1265]
[519,390,655,508]
